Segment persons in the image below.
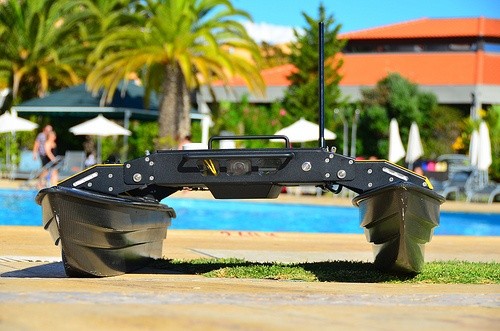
[180,135,192,151]
[33,125,57,185]
[83,141,97,169]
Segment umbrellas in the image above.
[0,111,38,166]
[70,114,132,163]
[269,117,336,149]
[469,122,492,190]
[406,121,423,170]
[388,117,405,164]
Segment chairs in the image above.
[58,152,89,182]
[430,154,500,204]
